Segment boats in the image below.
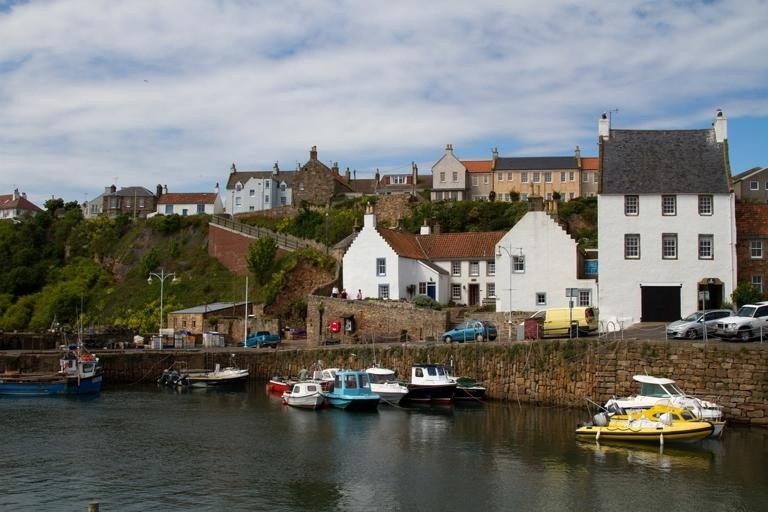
[574,399,714,445]
[601,372,724,422]
[259,361,490,412]
[154,360,250,389]
[0,295,106,397]
[706,419,729,437]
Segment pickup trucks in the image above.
[242,330,281,349]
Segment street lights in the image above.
[147,269,179,350]
[492,244,527,339]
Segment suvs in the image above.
[713,300,767,343]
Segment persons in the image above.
[331,283,340,297]
[339,289,348,299]
[297,366,311,381]
[356,289,362,300]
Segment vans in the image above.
[518,304,599,339]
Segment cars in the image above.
[440,316,498,344]
[667,308,734,340]
